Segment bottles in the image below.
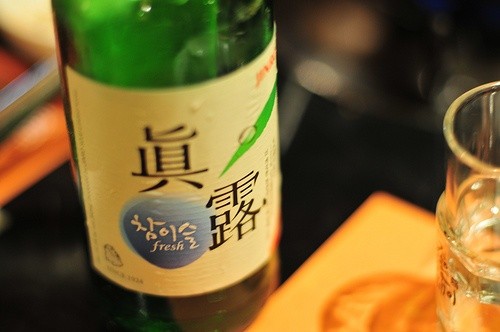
[46,0,287,324]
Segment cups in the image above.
[429,78,500,332]
